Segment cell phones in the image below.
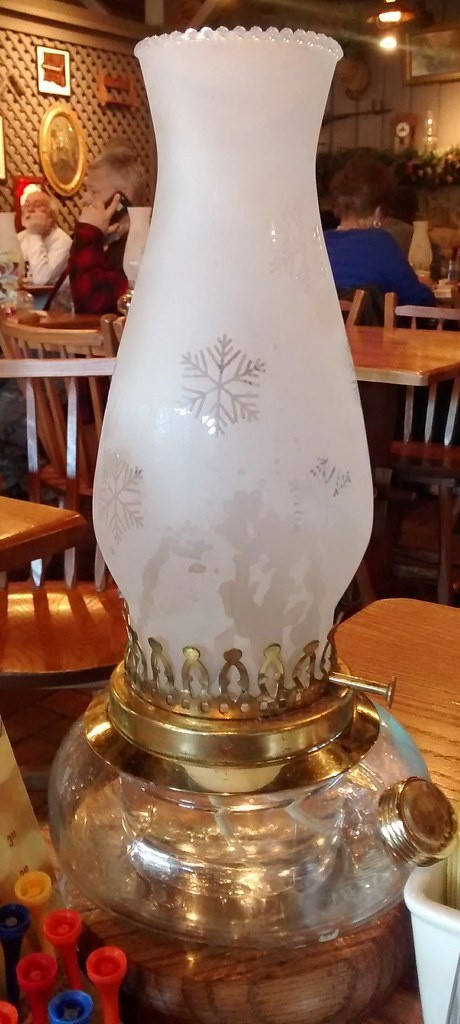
[104,190,133,226]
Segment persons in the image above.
[323,158,451,331]
[69,147,145,323]
[16,191,75,313]
[381,187,441,280]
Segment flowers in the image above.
[391,146,460,186]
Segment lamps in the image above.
[423,109,437,153]
[117,207,152,316]
[49,23,454,1024]
[408,221,433,280]
[0,212,34,315]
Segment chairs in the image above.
[1,289,460,820]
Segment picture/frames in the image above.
[36,45,71,96]
[38,104,87,196]
[404,22,460,85]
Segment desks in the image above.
[347,326,460,576]
[20,310,104,356]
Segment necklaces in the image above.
[337,225,356,231]
